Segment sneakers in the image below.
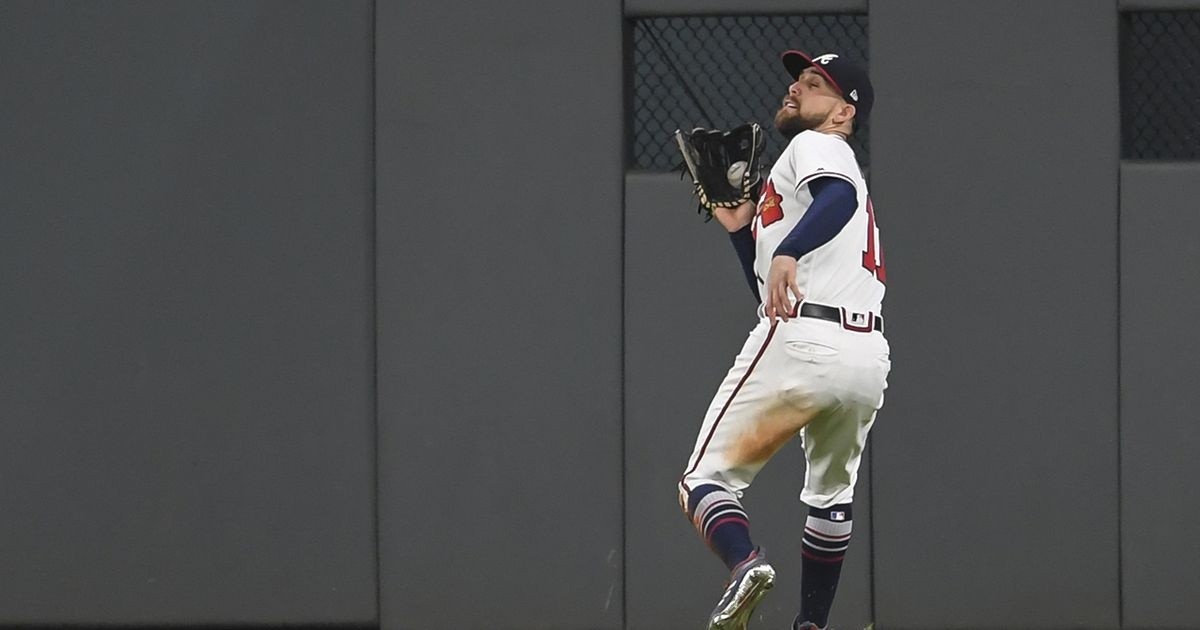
[791,612,830,630]
[706,550,776,630]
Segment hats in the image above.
[780,49,875,131]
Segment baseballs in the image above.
[726,160,748,190]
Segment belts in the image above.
[766,301,882,331]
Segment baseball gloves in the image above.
[674,119,768,224]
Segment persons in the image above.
[677,50,891,630]
